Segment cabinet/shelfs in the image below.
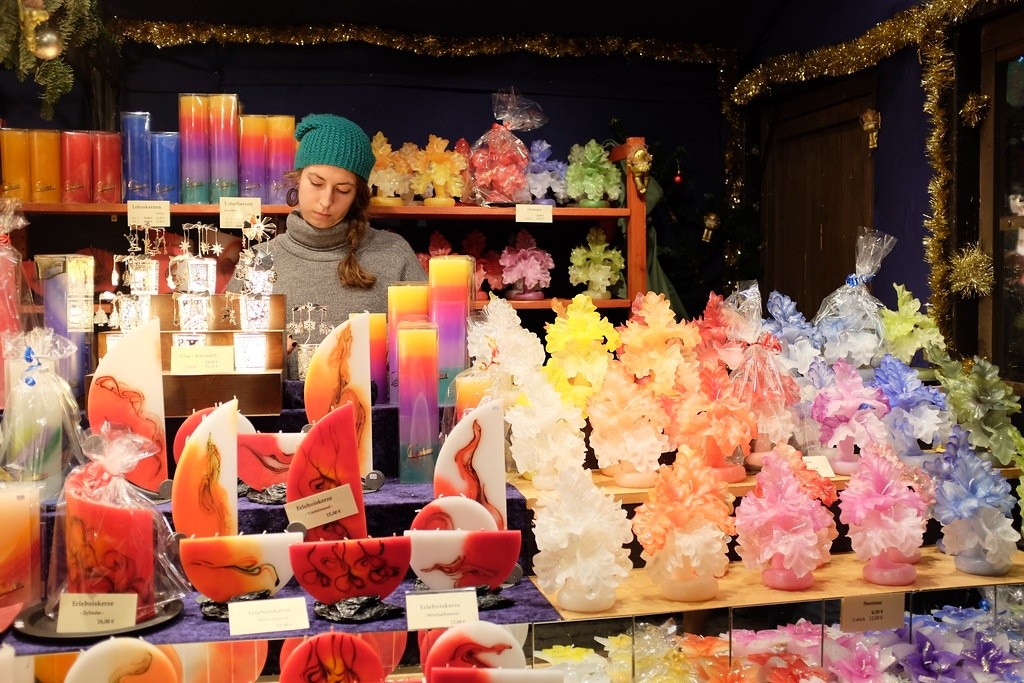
[0,135,1024,683]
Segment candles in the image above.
[0,91,301,205]
[394,314,439,482]
[384,279,432,405]
[369,312,387,406]
[428,255,474,407]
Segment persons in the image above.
[223,115,428,380]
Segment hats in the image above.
[294,113,376,182]
